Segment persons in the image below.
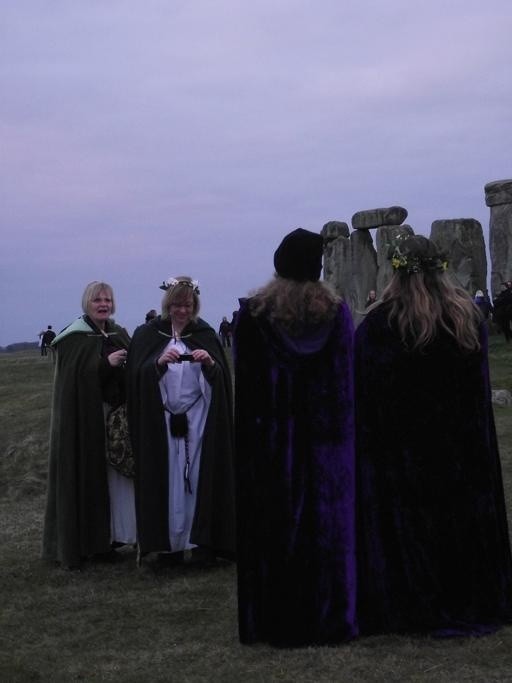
[226,227,360,647]
[124,276,233,566]
[364,289,378,309]
[43,325,57,364]
[492,282,511,337]
[43,280,139,569]
[354,233,511,638]
[474,289,489,319]
[37,327,47,356]
[219,316,232,348]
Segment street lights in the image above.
[168,411,189,438]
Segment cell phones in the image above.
[178,354,194,363]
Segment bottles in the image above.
[273,226,324,281]
